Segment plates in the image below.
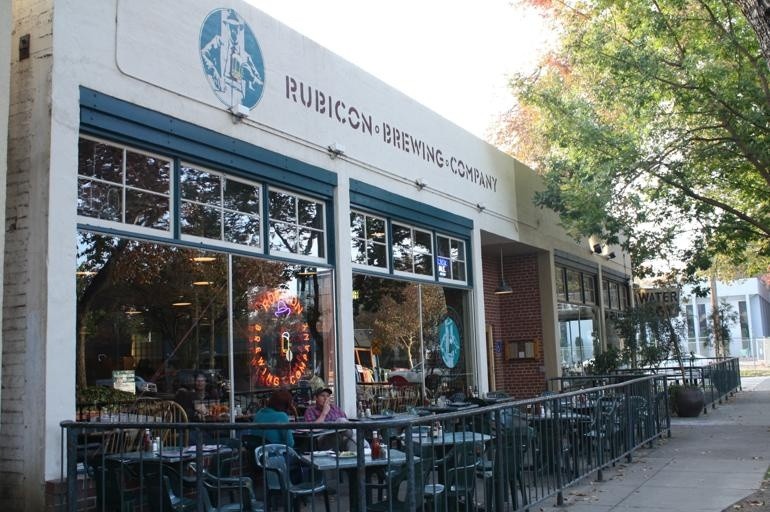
[312,447,371,458]
[348,414,395,421]
[156,443,223,459]
[294,429,324,434]
[448,402,471,407]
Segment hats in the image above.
[316,386,332,395]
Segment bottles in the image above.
[429,389,436,406]
[534,394,545,417]
[468,384,478,398]
[144,429,160,452]
[380,443,388,460]
[236,404,242,416]
[358,401,371,418]
[438,425,443,436]
[431,412,438,437]
[371,430,380,459]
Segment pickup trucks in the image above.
[97,374,160,396]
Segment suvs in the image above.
[387,359,448,387]
[170,369,231,396]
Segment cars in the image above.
[648,353,714,369]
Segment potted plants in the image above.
[589,298,741,417]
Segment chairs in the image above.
[69,362,672,512]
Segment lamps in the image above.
[592,242,604,256]
[607,251,618,260]
[330,141,345,155]
[494,243,513,295]
[478,201,487,211]
[231,101,249,118]
[416,176,428,187]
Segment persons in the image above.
[135,359,154,379]
[169,370,352,476]
